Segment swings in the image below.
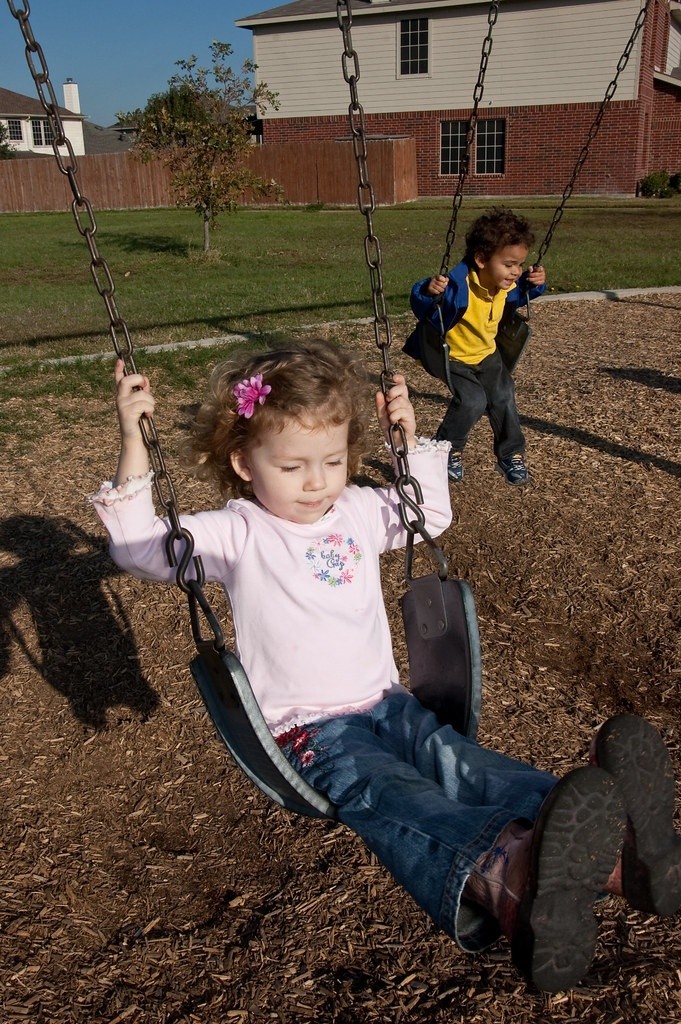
[2,0,486,822]
[398,1,656,402]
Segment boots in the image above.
[591,713,681,916]
[464,767,627,994]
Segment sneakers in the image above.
[495,457,527,486]
[448,452,464,482]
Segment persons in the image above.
[92,341,680,991]
[403,205,546,487]
